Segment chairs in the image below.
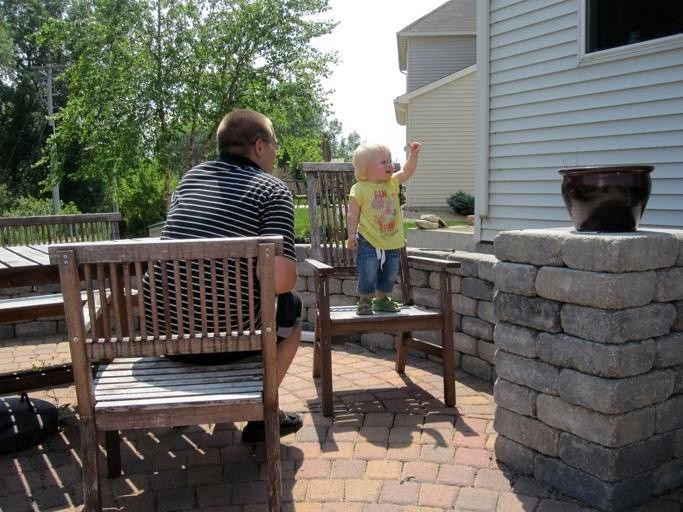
[301,161,461,417]
[0,213,285,512]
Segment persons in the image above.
[346,141,422,315]
[141,109,302,444]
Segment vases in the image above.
[557,164,655,233]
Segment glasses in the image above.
[253,136,282,151]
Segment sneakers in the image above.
[357,299,373,315]
[374,297,401,312]
[241,408,303,441]
